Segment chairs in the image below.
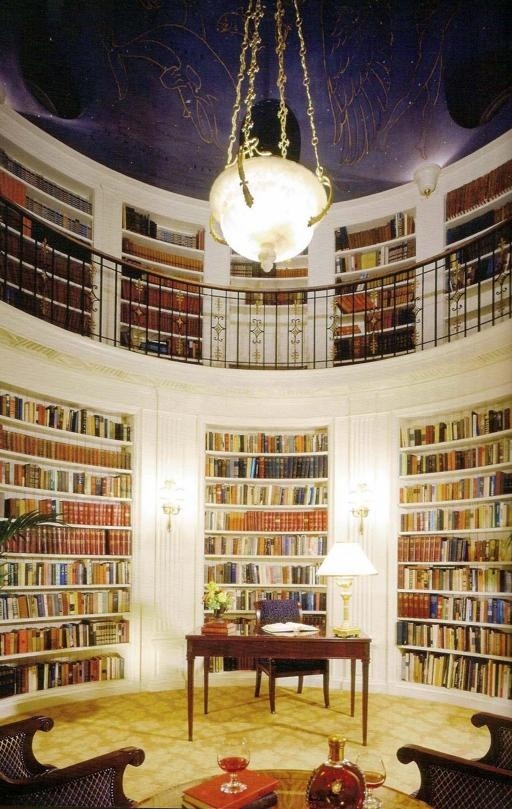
[396,709,511,809]
[0,716,146,809]
[253,600,330,714]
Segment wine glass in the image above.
[353,750,387,809]
[215,734,251,795]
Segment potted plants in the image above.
[199,580,233,637]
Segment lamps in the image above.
[316,540,378,638]
[413,84,442,198]
[206,0,334,273]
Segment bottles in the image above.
[304,734,366,809]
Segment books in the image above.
[444,159,512,294]
[203,431,329,673]
[119,202,206,360]
[332,211,416,367]
[0,395,132,699]
[396,407,512,700]
[0,147,94,338]
[229,246,308,306]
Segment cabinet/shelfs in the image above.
[224,240,309,313]
[0,135,105,340]
[196,418,337,687]
[0,380,141,721]
[115,200,207,365]
[442,158,510,319]
[334,207,418,366]
[386,389,511,717]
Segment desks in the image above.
[185,632,372,746]
[130,769,436,809]
